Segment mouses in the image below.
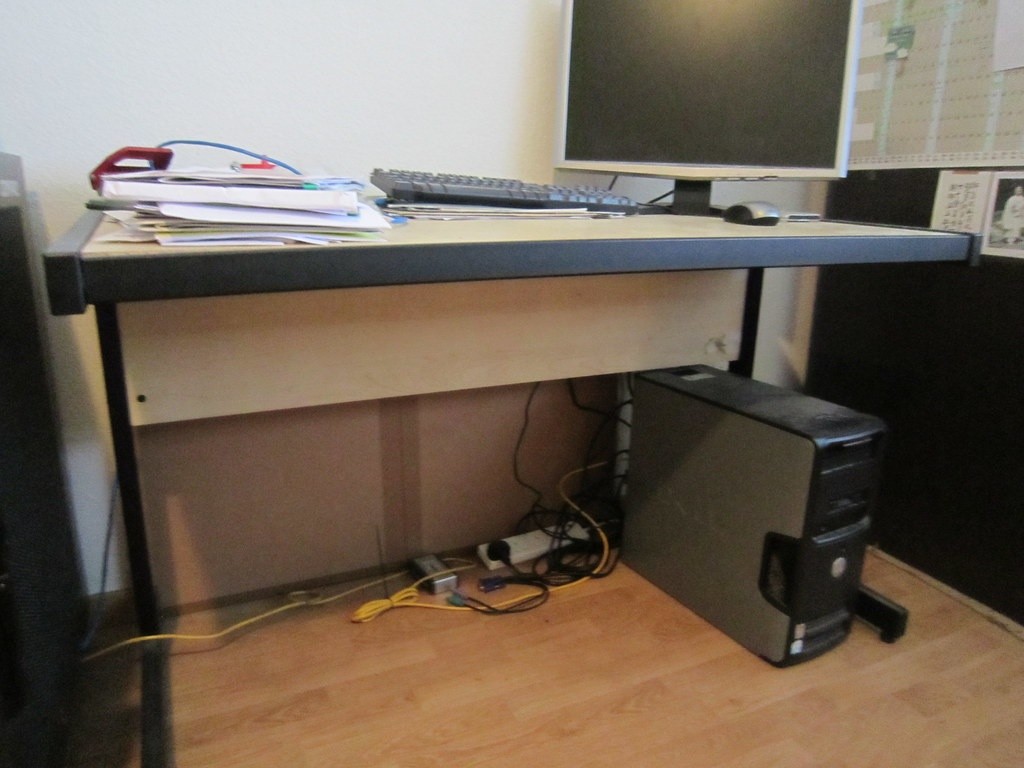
[724,201,780,227]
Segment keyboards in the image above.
[369,167,640,216]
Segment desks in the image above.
[37,206,984,768]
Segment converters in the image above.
[409,553,459,593]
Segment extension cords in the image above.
[478,518,588,570]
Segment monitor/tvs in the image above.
[554,0,864,218]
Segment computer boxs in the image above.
[620,366,887,671]
